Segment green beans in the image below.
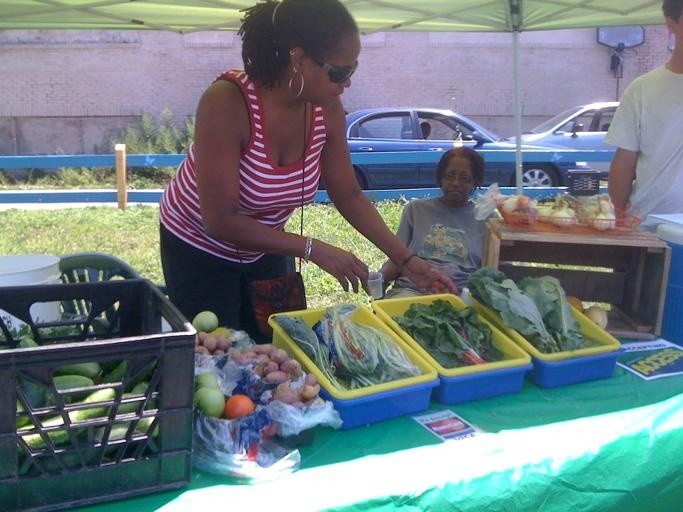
[290,303,422,392]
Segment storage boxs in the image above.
[370,294,533,406]
[469,287,624,389]
[0,280,198,512]
[480,216,671,339]
[268,305,439,432]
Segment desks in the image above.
[0,339,683,511]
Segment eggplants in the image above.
[3,338,159,474]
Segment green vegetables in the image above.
[391,299,503,369]
[467,265,584,353]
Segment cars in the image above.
[319,107,577,189]
[507,101,621,180]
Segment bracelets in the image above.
[305,236,312,264]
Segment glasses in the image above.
[297,44,359,83]
[442,172,478,184]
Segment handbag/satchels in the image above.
[226,99,310,338]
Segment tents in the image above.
[0,0,665,204]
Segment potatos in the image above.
[196,328,227,357]
[231,343,321,408]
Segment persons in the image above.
[379,146,503,298]
[159,1,458,341]
[420,121,431,140]
[602,0,683,229]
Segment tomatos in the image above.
[225,395,253,418]
[192,385,225,419]
[193,372,218,389]
